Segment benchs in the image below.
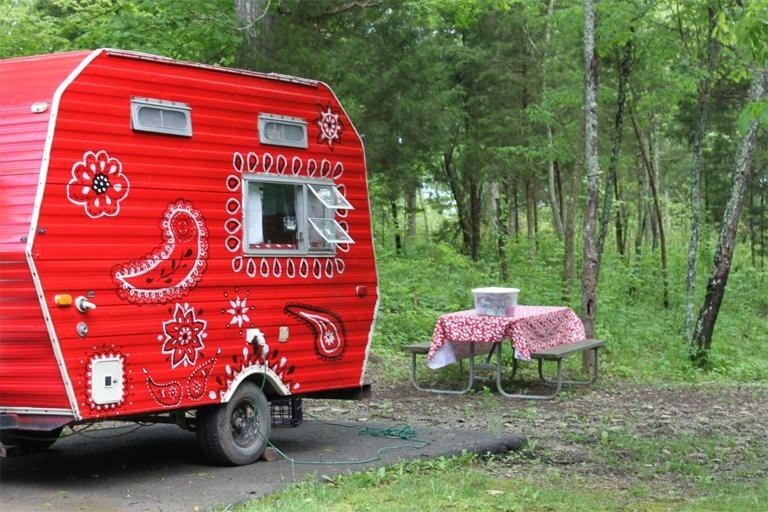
[530,342,604,400]
[401,342,432,395]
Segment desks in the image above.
[426,306,586,400]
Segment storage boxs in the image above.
[471,287,520,317]
